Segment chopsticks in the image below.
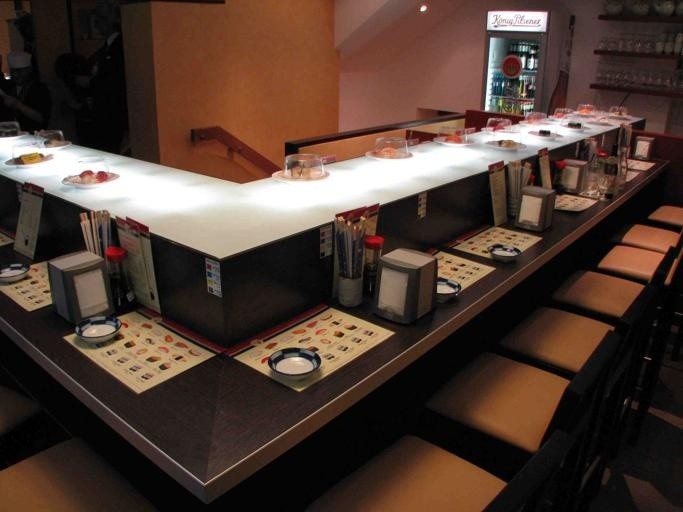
[79,210,112,270]
[333,214,365,278]
[507,160,531,195]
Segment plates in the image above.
[432,136,482,147]
[74,315,123,344]
[528,131,564,140]
[62,172,122,189]
[591,120,609,124]
[480,127,521,134]
[267,346,324,382]
[486,242,523,263]
[433,276,462,305]
[559,125,591,132]
[31,140,73,151]
[271,169,331,184]
[519,120,552,129]
[365,151,415,163]
[0,151,54,167]
[1,264,31,284]
[0,132,30,142]
[484,141,528,151]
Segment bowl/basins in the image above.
[439,126,467,144]
[76,155,111,171]
[487,117,512,130]
[0,121,20,136]
[553,108,573,120]
[37,129,64,147]
[283,154,325,179]
[526,111,548,125]
[12,143,40,160]
[577,103,595,114]
[374,138,408,158]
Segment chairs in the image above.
[0,438,153,512]
[622,224,682,258]
[310,425,575,512]
[595,233,683,283]
[649,204,682,228]
[554,244,677,321]
[499,283,656,372]
[0,382,43,439]
[428,330,620,453]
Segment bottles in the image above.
[528,174,535,189]
[490,43,535,119]
[364,235,383,298]
[102,245,137,314]
[554,160,567,192]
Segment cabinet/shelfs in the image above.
[587,12,683,97]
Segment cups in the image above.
[581,162,601,196]
[507,195,520,217]
[338,276,363,307]
